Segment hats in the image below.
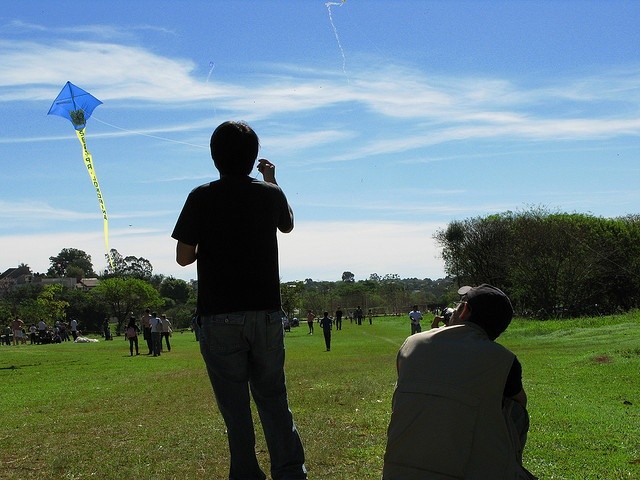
[458,284,513,332]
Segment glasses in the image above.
[453,300,465,308]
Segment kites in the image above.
[48,80,116,269]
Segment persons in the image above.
[140,308,153,355]
[4,323,12,344]
[306,309,316,335]
[384,310,387,317]
[409,304,423,335]
[12,316,25,345]
[320,311,332,352]
[382,284,538,480]
[427,308,430,312]
[29,323,37,345]
[124,323,129,341]
[345,315,348,320]
[160,313,171,352]
[107,327,111,340]
[367,309,374,325]
[22,329,28,344]
[189,316,199,341]
[348,310,353,323]
[171,121,309,480]
[38,315,83,345]
[103,317,109,341]
[127,316,141,356]
[149,312,162,357]
[334,305,343,331]
[353,308,357,324]
[357,305,362,325]
